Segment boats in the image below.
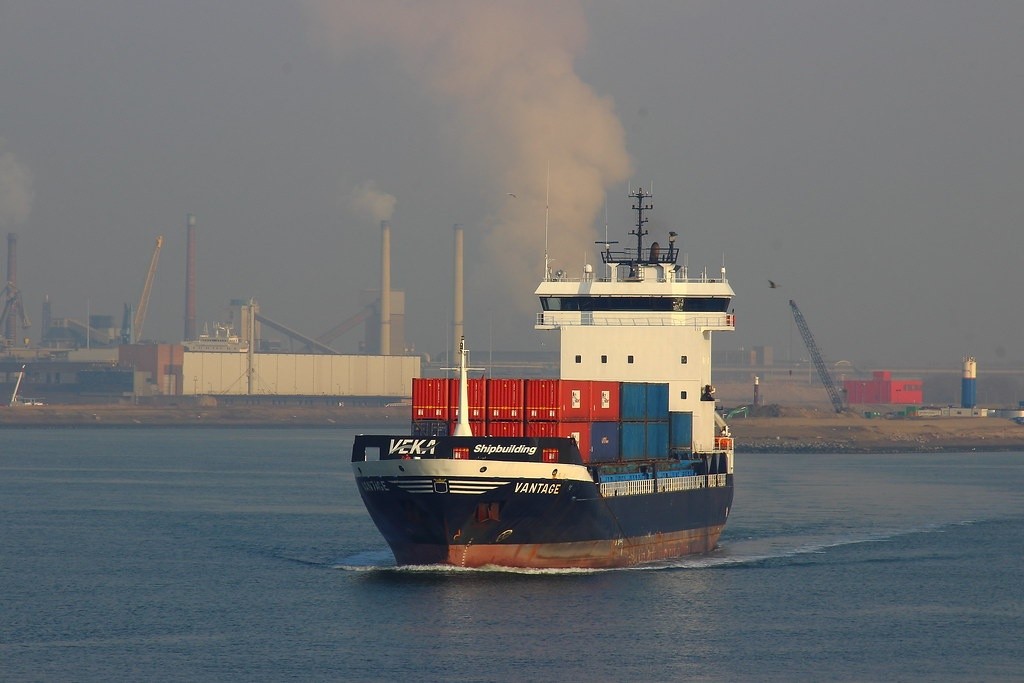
[351,158,737,573]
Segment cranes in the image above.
[788,299,844,414]
[125,234,164,345]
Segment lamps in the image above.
[669,230,678,242]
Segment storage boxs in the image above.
[526,378,558,422]
[488,378,525,422]
[647,383,671,421]
[450,421,486,437]
[620,381,647,422]
[449,378,486,421]
[525,422,557,438]
[411,378,450,421]
[646,421,670,460]
[557,379,621,423]
[411,420,449,436]
[670,411,693,450]
[557,422,621,464]
[621,422,647,462]
[488,422,524,437]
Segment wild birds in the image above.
[768,280,781,288]
